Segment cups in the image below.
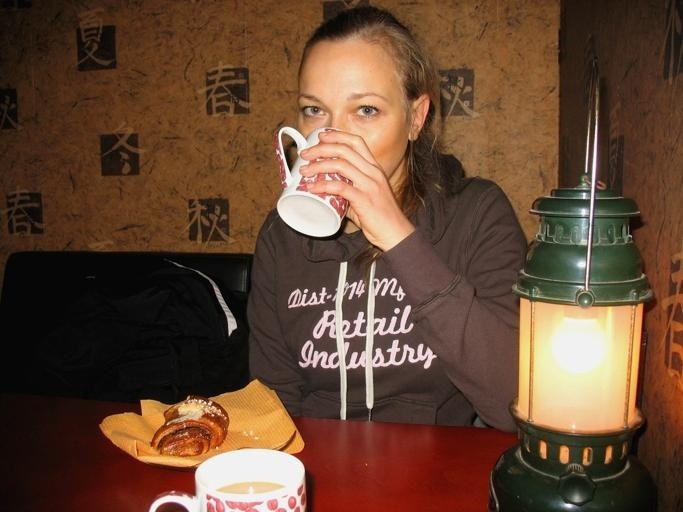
[149,447,306,512]
[272,125,349,239]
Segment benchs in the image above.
[1,249,255,405]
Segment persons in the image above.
[245,6,530,435]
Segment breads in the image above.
[152,393,229,457]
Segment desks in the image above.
[0,387,517,512]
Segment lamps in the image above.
[487,32,672,511]
